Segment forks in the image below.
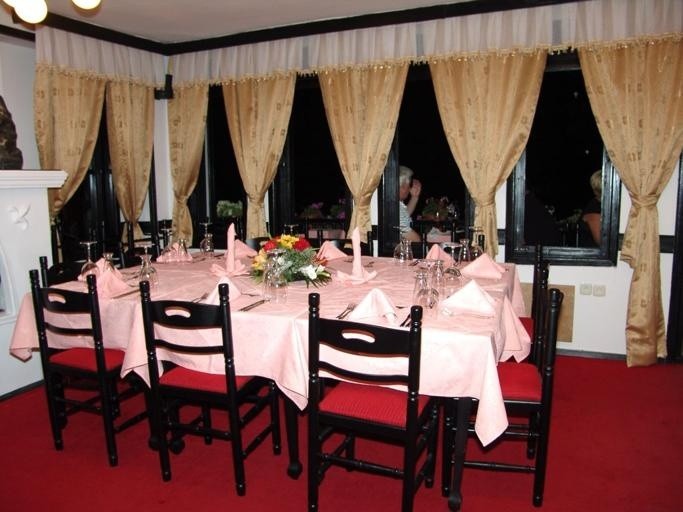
[191,292,210,303]
[333,301,359,321]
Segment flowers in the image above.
[215,200,243,218]
[296,197,348,219]
[416,196,454,221]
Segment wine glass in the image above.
[393,224,414,266]
[159,221,216,263]
[408,225,486,309]
[138,254,159,289]
[262,248,288,307]
[78,241,116,282]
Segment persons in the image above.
[577,170,601,248]
[398,165,424,242]
